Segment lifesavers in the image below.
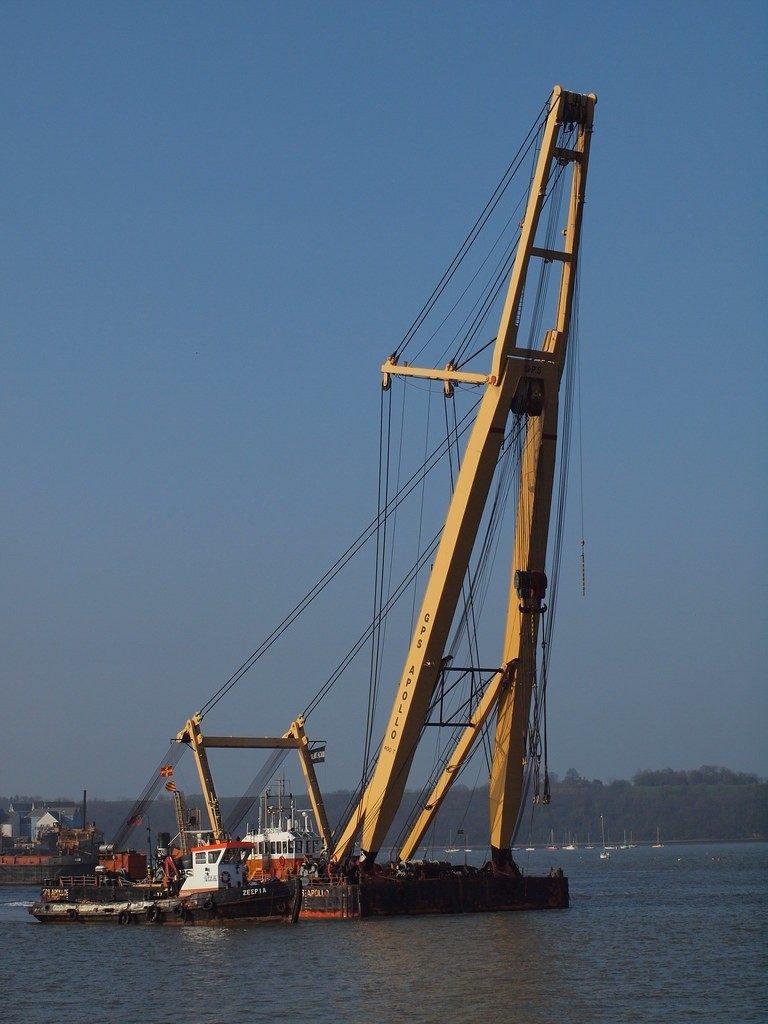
[172,905,184,917]
[279,856,285,866]
[69,911,77,921]
[146,906,160,922]
[117,910,130,924]
[202,901,213,911]
[275,901,289,914]
[220,871,230,883]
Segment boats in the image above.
[510,847,520,851]
[28,837,365,927]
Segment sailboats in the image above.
[561,831,577,851]
[525,834,536,851]
[599,815,611,860]
[585,833,596,850]
[620,830,638,850]
[603,826,618,850]
[544,828,559,851]
[463,835,473,853]
[442,828,461,853]
[651,825,665,848]
